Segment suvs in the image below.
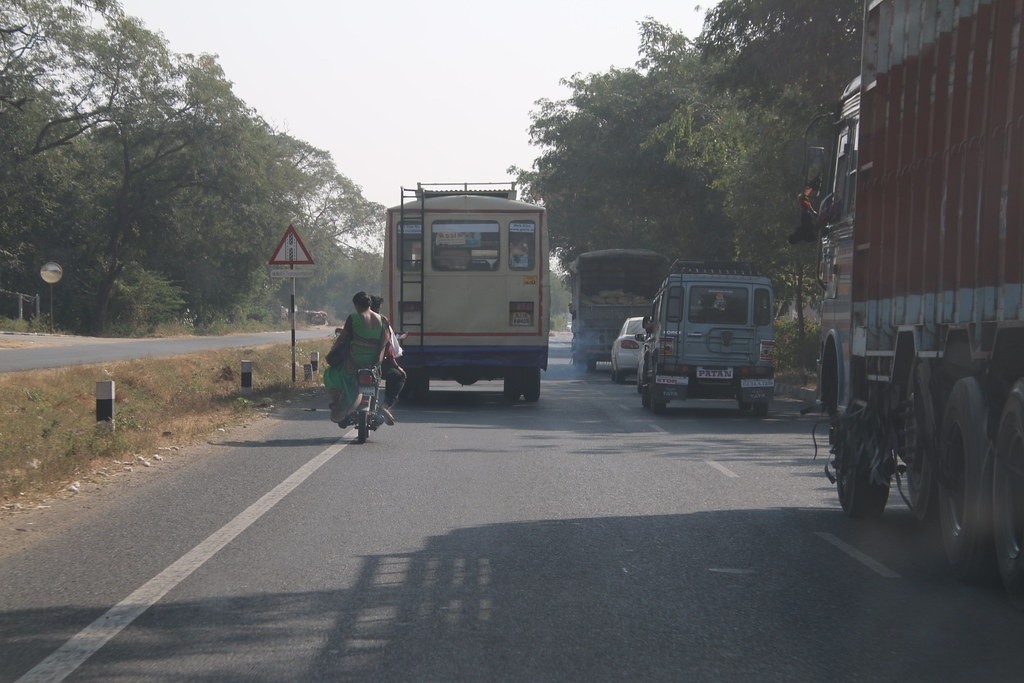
[639,261,774,418]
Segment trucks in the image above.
[569,249,686,374]
[801,1,1024,607]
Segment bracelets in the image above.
[396,365,400,368]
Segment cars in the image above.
[610,316,646,382]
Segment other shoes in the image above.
[330,408,348,422]
[378,405,395,426]
[347,393,362,416]
[328,403,337,410]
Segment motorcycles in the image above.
[335,327,409,442]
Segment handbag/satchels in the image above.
[325,315,352,367]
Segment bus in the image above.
[380,179,551,401]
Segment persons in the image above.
[323,292,407,429]
